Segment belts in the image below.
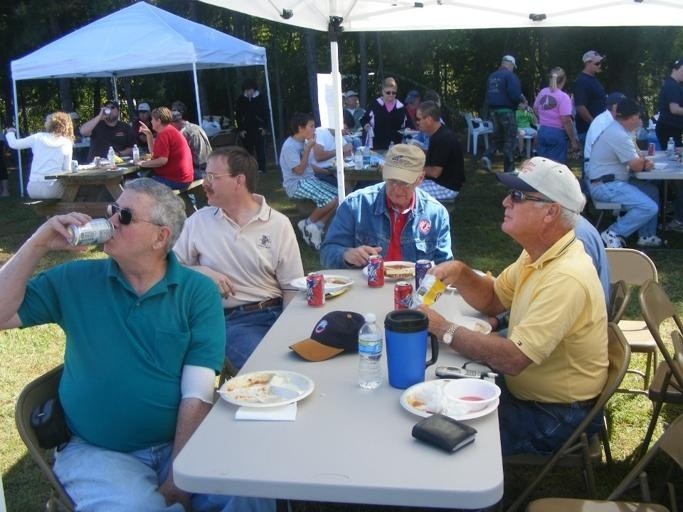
[223,296,283,315]
[583,157,590,164]
[589,173,616,185]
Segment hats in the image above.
[380,142,427,185]
[495,155,587,214]
[137,102,150,112]
[502,54,518,70]
[344,90,359,98]
[403,90,420,104]
[170,110,182,121]
[526,396,600,410]
[604,91,648,118]
[287,309,366,362]
[104,100,120,111]
[582,49,606,63]
[69,112,80,121]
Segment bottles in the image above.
[352,143,370,170]
[667,137,675,158]
[132,144,138,163]
[388,140,393,150]
[356,313,382,389]
[107,146,115,170]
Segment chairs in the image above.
[636,278,682,462]
[597,248,660,394]
[12,362,83,512]
[524,411,682,512]
[497,319,632,512]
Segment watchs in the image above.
[440,323,461,346]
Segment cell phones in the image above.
[436,367,483,380]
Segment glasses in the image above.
[508,188,553,204]
[106,202,163,227]
[383,90,397,95]
[202,172,233,180]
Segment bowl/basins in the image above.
[441,377,500,411]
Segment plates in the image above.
[216,370,313,409]
[288,275,353,290]
[399,378,500,421]
[361,261,485,291]
[445,315,492,336]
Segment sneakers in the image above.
[600,228,627,248]
[503,167,519,175]
[480,155,493,172]
[297,218,312,245]
[636,234,669,248]
[305,221,324,251]
[658,219,683,232]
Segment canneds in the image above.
[306,272,325,307]
[415,259,432,292]
[65,217,116,247]
[104,108,112,115]
[648,143,655,156]
[394,281,413,311]
[94,156,101,168]
[367,255,384,288]
[70,160,78,173]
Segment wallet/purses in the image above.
[413,413,477,452]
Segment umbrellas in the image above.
[197,0,683,220]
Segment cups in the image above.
[383,308,438,389]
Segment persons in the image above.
[229,79,269,176]
[315,141,454,273]
[168,144,307,372]
[486,212,611,342]
[0,98,214,225]
[407,154,614,512]
[278,47,682,255]
[0,173,281,511]
